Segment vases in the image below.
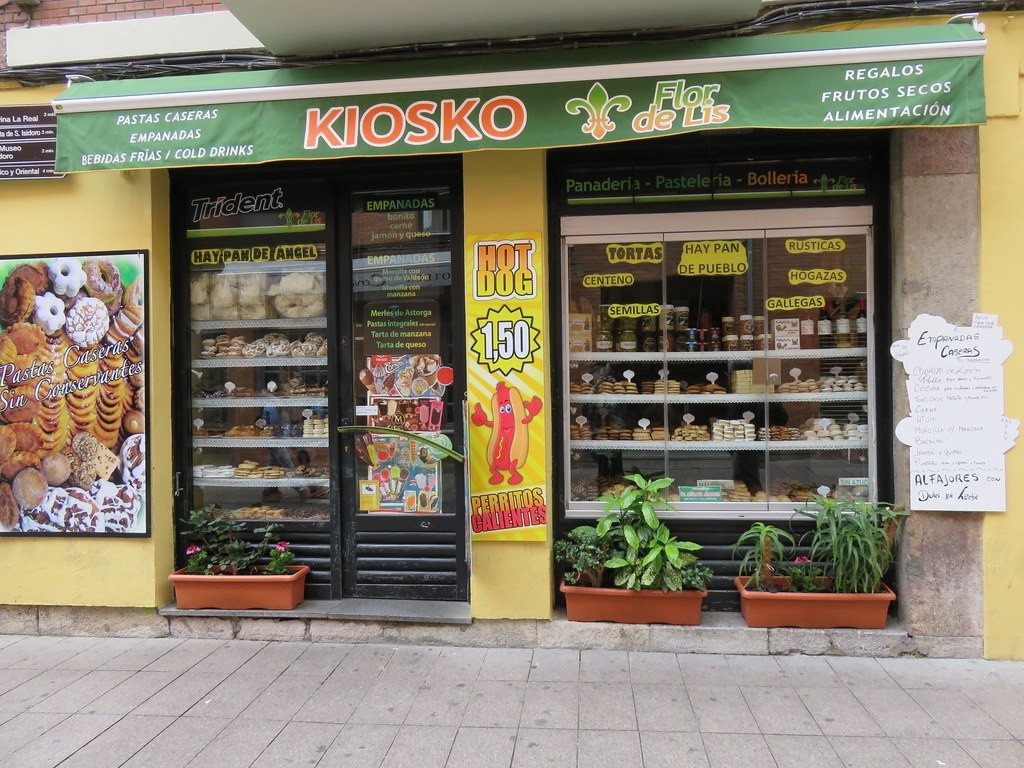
[168,566,309,610]
[735,576,896,628]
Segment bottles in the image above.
[856,296,867,347]
[818,306,834,348]
[835,301,852,348]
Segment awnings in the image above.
[52,12,988,174]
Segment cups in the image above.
[404,491,416,513]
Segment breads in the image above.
[569,374,869,502]
[186,270,329,519]
[0,256,145,531]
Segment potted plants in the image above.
[553,473,713,626]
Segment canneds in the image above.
[594,304,773,352]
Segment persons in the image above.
[260,370,329,504]
[729,365,789,498]
[581,366,629,495]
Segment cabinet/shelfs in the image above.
[190,262,331,523]
[561,226,877,511]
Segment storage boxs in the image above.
[770,319,801,350]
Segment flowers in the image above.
[731,494,910,593]
[179,503,295,576]
